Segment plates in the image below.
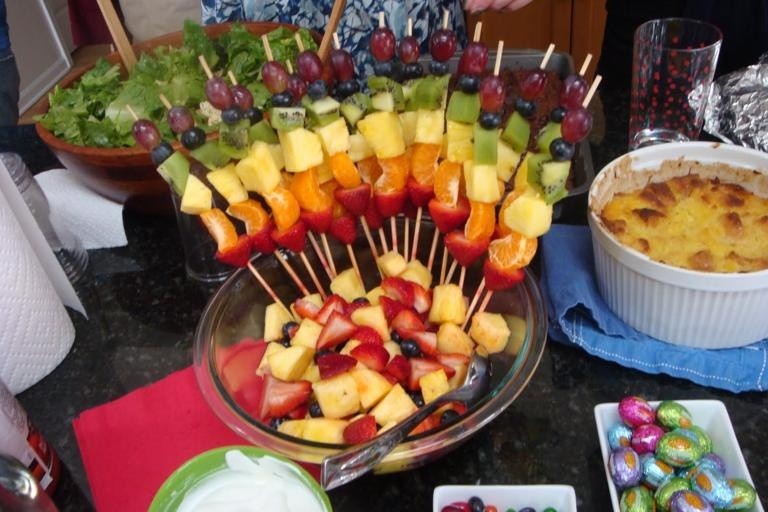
[592,397,765,511]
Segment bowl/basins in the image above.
[33,21,329,206]
[585,142,767,352]
[189,210,550,476]
[145,443,335,512]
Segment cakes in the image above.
[604,174,768,273]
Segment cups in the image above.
[628,16,722,153]
[1,149,89,282]
[168,159,237,284]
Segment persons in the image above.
[591,2,768,135]
[197,1,533,70]
[1,1,23,155]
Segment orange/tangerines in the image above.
[201,140,537,272]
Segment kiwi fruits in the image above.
[153,71,573,207]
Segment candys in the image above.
[607,394,756,512]
[441,494,558,511]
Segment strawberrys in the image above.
[214,178,526,449]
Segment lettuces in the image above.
[30,18,319,147]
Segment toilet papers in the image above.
[0,159,89,398]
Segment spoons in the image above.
[321,352,490,495]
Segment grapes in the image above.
[132,26,594,166]
